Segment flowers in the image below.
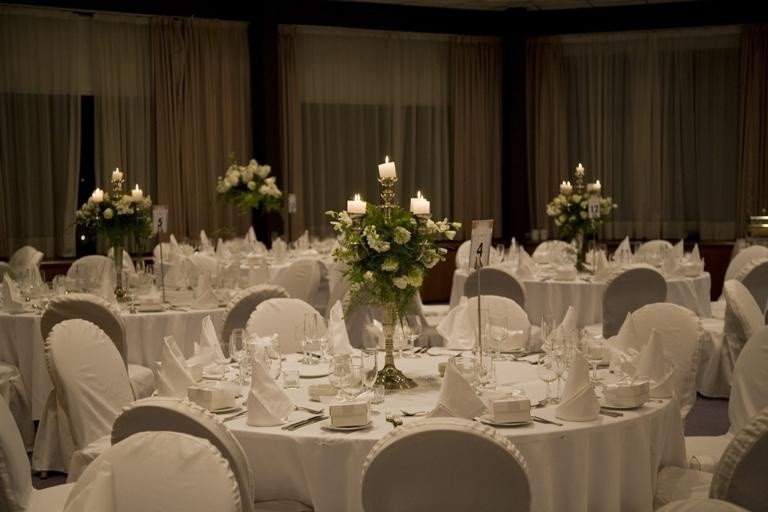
[546,192,618,239]
[75,193,152,244]
[214,152,283,215]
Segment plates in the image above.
[600,401,643,408]
[297,365,333,377]
[213,403,240,413]
[321,420,372,430]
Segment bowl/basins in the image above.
[310,383,336,400]
[438,362,448,377]
[481,416,528,426]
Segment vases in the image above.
[575,233,592,272]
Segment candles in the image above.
[560,181,566,192]
[132,183,143,201]
[576,162,584,175]
[413,195,430,215]
[111,167,122,181]
[566,180,572,192]
[594,179,601,190]
[347,191,365,213]
[91,187,103,201]
[378,155,397,178]
[410,190,426,212]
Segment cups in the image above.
[283,370,298,388]
[456,358,497,390]
[371,385,384,404]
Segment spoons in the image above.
[400,408,430,416]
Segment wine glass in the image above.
[538,312,568,406]
[162,233,335,268]
[393,312,423,360]
[228,328,249,389]
[330,347,380,406]
[295,311,332,366]
[12,259,233,314]
[587,342,604,381]
[490,242,700,280]
[488,318,508,361]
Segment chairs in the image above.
[324,203,462,329]
